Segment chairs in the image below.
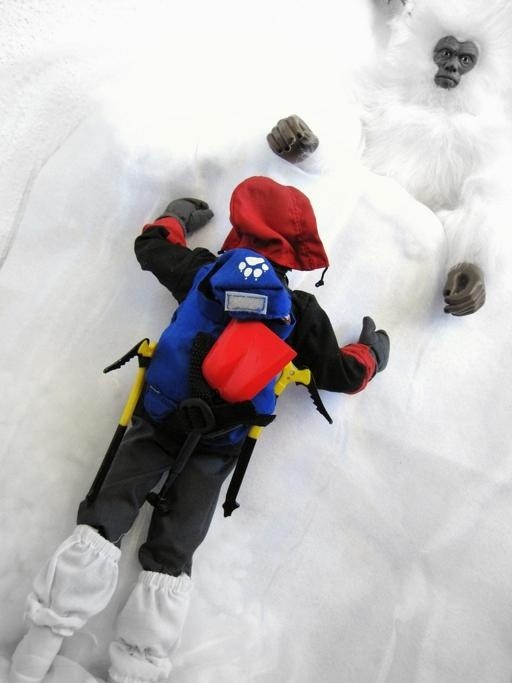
[6,626,64,683]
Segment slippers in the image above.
[143,248,297,439]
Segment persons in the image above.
[267,35,486,317]
[9,177,392,683]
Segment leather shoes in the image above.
[358,317,389,372]
[156,198,214,239]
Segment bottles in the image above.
[218,176,329,271]
